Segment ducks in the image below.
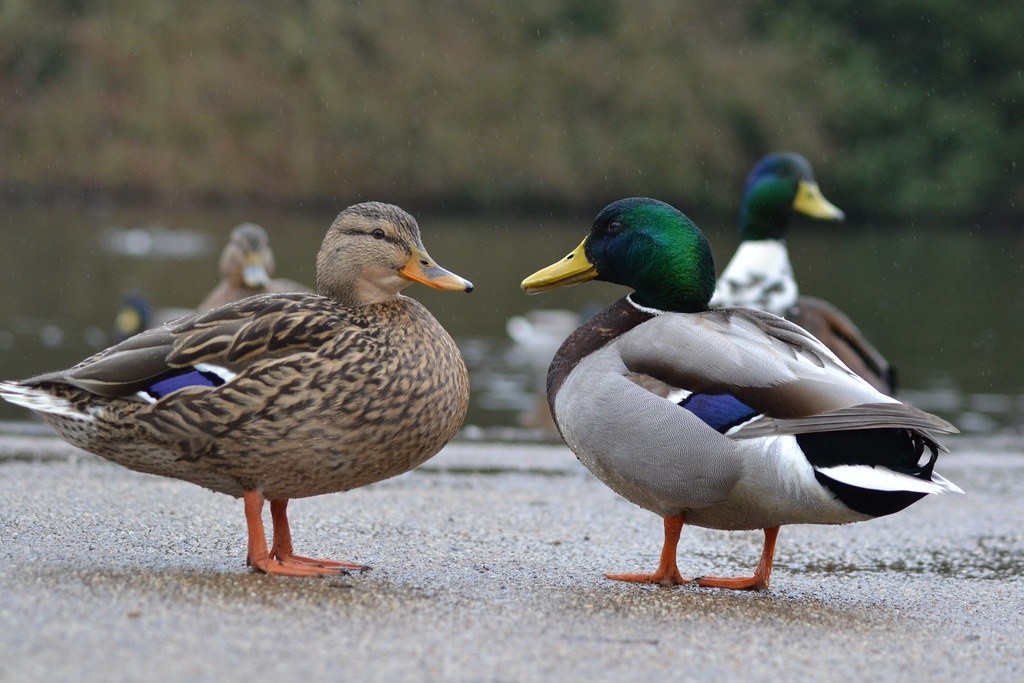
[704,151,898,399]
[520,197,964,590]
[0,203,474,577]
